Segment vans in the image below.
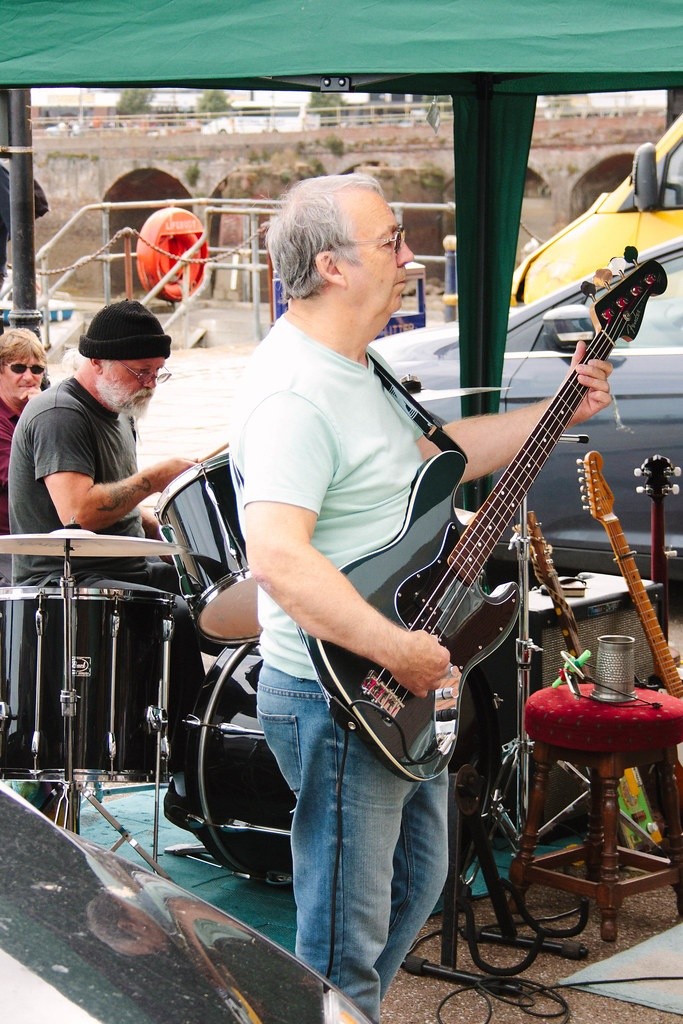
[509,109,683,310]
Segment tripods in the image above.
[37,539,175,883]
[458,487,668,904]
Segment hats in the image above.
[78,298,172,360]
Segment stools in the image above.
[508,683,683,943]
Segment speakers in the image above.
[479,571,666,827]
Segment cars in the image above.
[370,232,683,583]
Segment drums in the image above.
[183,643,297,881]
[154,445,272,647]
[2,585,181,787]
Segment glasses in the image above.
[2,362,45,374]
[115,360,172,384]
[328,224,403,251]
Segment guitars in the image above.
[318,243,670,782]
[514,508,591,685]
[577,451,683,707]
[634,455,681,638]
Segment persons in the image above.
[227,175,614,1024]
[85,881,254,958]
[8,300,207,834]
[0,327,46,537]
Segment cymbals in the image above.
[0,525,191,560]
